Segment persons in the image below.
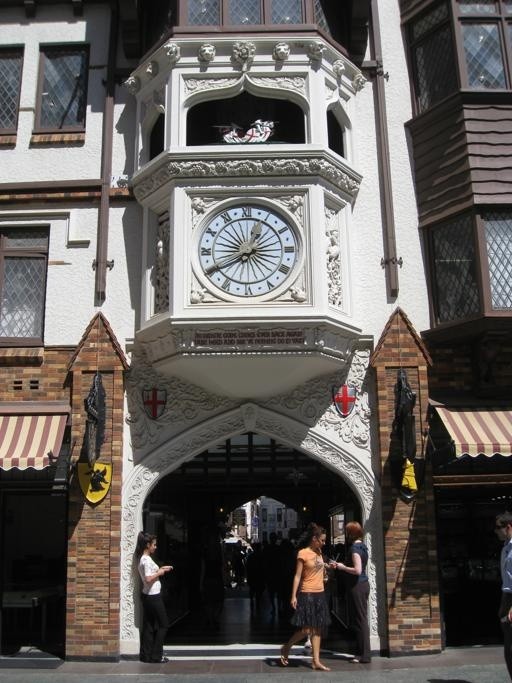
[493,506,512,682]
[326,520,373,664]
[279,521,333,672]
[134,531,174,664]
[189,525,299,626]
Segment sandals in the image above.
[279,645,289,666]
[312,661,330,671]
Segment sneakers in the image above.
[349,657,371,664]
[142,657,169,664]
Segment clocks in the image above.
[190,197,307,302]
